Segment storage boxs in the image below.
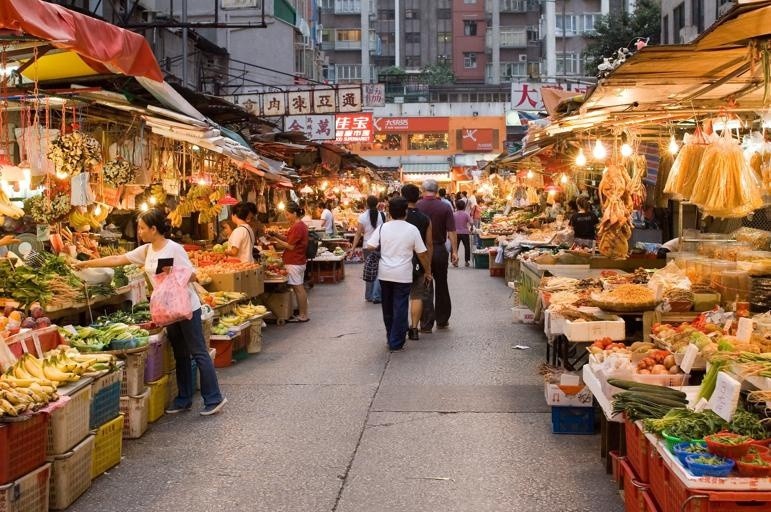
[544,380,593,407]
[206,267,266,298]
[263,291,294,320]
[692,291,721,311]
[544,307,626,342]
[550,405,595,435]
[634,373,692,386]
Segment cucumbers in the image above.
[606,375,691,420]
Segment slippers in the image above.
[287,315,309,322]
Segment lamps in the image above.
[300,179,314,193]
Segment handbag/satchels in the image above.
[143,244,153,293]
[307,230,321,259]
[362,250,379,282]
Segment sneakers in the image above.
[200,396,227,416]
[369,299,381,304]
[165,402,192,414]
[389,348,408,353]
[420,321,449,334]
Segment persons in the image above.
[72,209,227,415]
[246,202,257,236]
[317,203,333,238]
[224,202,255,265]
[567,195,599,249]
[349,179,484,353]
[267,201,310,322]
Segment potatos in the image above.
[188,248,261,274]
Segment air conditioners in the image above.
[519,55,528,62]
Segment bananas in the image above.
[0,186,26,227]
[67,203,110,229]
[0,348,99,417]
[150,182,225,229]
[219,299,267,328]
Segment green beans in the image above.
[684,433,770,470]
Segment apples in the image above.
[0,305,51,339]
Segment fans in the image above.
[10,233,44,264]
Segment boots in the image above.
[408,325,418,340]
